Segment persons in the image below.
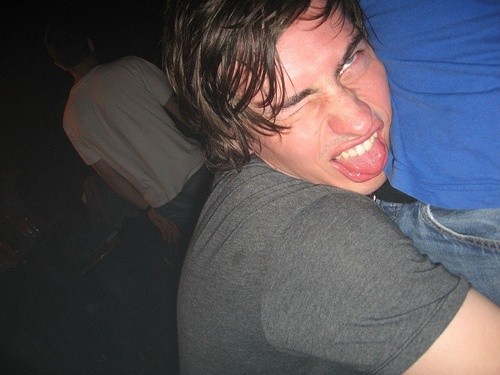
[348,0,500,304]
[42,23,209,247]
[157,0,500,375]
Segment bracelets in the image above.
[137,204,152,217]
